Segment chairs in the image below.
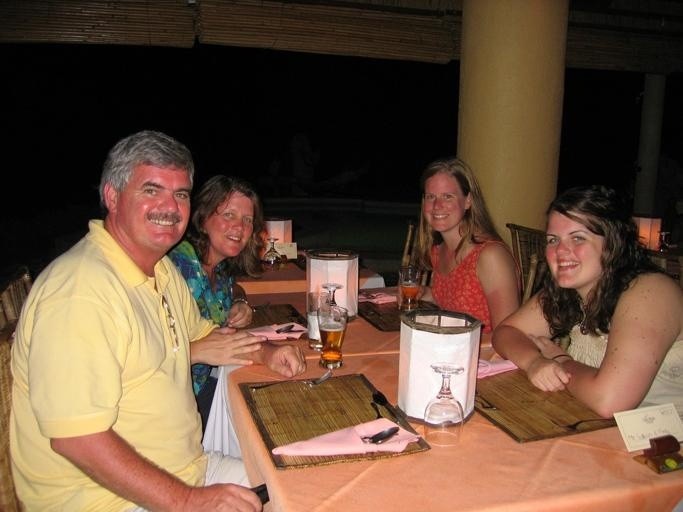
[3,217,683,511]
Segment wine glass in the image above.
[423,362,465,448]
[657,231,671,255]
[262,238,282,272]
[321,283,344,306]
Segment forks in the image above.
[249,367,332,391]
[560,418,615,431]
[372,389,411,433]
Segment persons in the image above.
[9,131,306,510]
[490,184,682,420]
[420,157,521,348]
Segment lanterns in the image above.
[306,250,359,322]
[398,304,483,427]
[261,214,294,263]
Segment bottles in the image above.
[317,282,349,369]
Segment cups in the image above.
[398,265,422,314]
[308,292,331,351]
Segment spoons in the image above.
[360,426,399,445]
[275,323,294,333]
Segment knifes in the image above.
[286,330,304,333]
